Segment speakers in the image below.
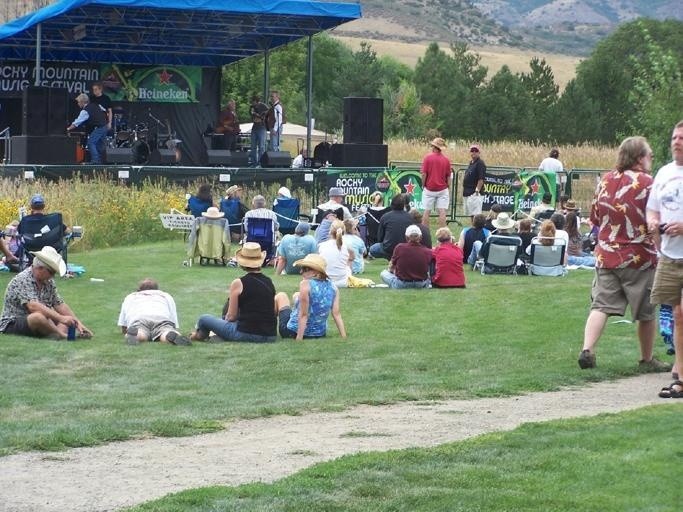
[105,148,134,165]
[22,85,46,136]
[260,150,292,168]
[11,134,76,164]
[205,149,232,167]
[47,86,68,135]
[148,148,177,166]
[330,144,389,168]
[343,97,384,144]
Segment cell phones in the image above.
[658,222,669,235]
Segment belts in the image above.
[399,277,427,283]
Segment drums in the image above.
[77,142,84,165]
[120,138,151,165]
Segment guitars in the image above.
[112,64,139,101]
[253,100,280,122]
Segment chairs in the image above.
[524,237,568,277]
[18,213,73,271]
[188,195,391,269]
[473,236,524,276]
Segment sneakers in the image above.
[577,350,596,371]
[125,326,139,346]
[6,257,24,264]
[165,331,210,347]
[637,357,670,369]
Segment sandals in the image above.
[657,380,683,399]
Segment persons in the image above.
[117,278,192,345]
[577,120,683,400]
[464,146,486,224]
[66,94,108,165]
[91,83,113,130]
[421,136,452,230]
[458,192,581,275]
[248,95,269,168]
[186,182,467,288]
[274,254,346,339]
[191,241,278,342]
[2,194,92,338]
[218,98,239,151]
[292,147,308,169]
[266,91,283,152]
[539,149,566,210]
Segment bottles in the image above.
[67,322,75,341]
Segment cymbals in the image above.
[138,129,151,138]
[118,130,130,140]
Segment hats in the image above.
[470,144,481,153]
[279,186,291,199]
[28,245,66,277]
[201,206,225,220]
[292,254,331,280]
[344,218,359,232]
[234,242,267,269]
[405,224,422,236]
[30,194,45,205]
[294,222,309,235]
[327,186,348,198]
[491,212,514,229]
[224,184,243,200]
[542,192,578,210]
[429,136,448,150]
